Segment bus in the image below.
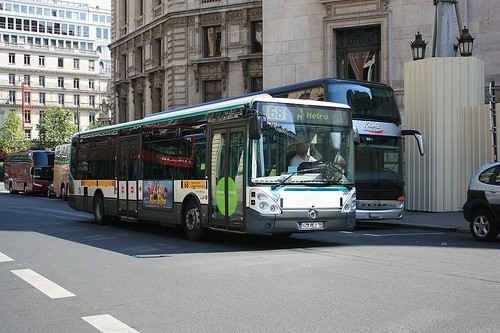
[4,150,53,195]
[69,94,356,242]
[54,144,70,198]
[144,79,425,222]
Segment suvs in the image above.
[462,161,500,240]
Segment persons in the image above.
[289,142,319,172]
